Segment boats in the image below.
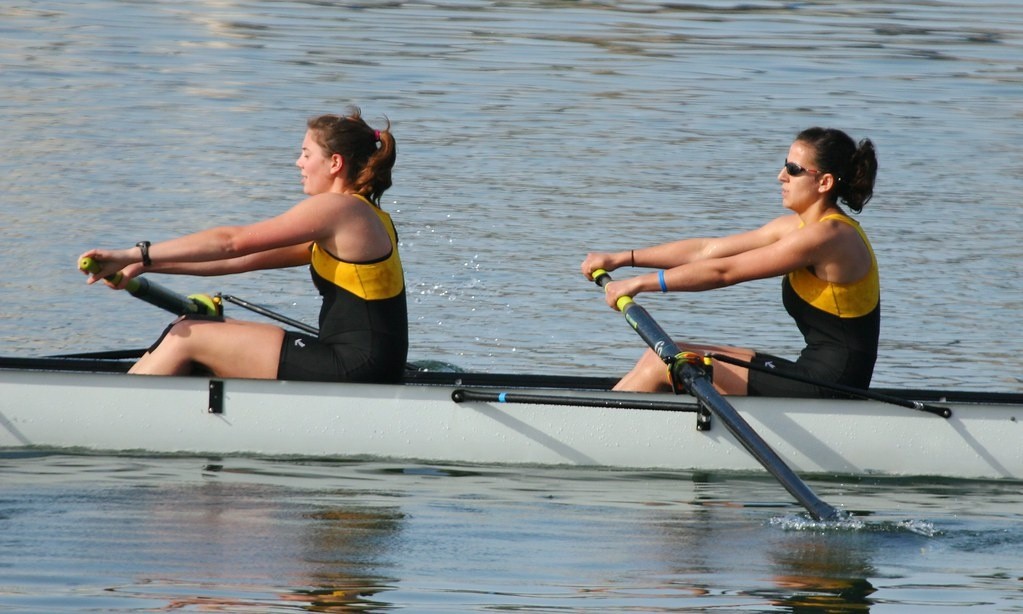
[1,347,1023,491]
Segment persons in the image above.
[583,128,881,398]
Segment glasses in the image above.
[784,158,823,177]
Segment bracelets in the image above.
[136,241,152,267]
[76,104,410,389]
[658,270,668,293]
[631,250,634,267]
